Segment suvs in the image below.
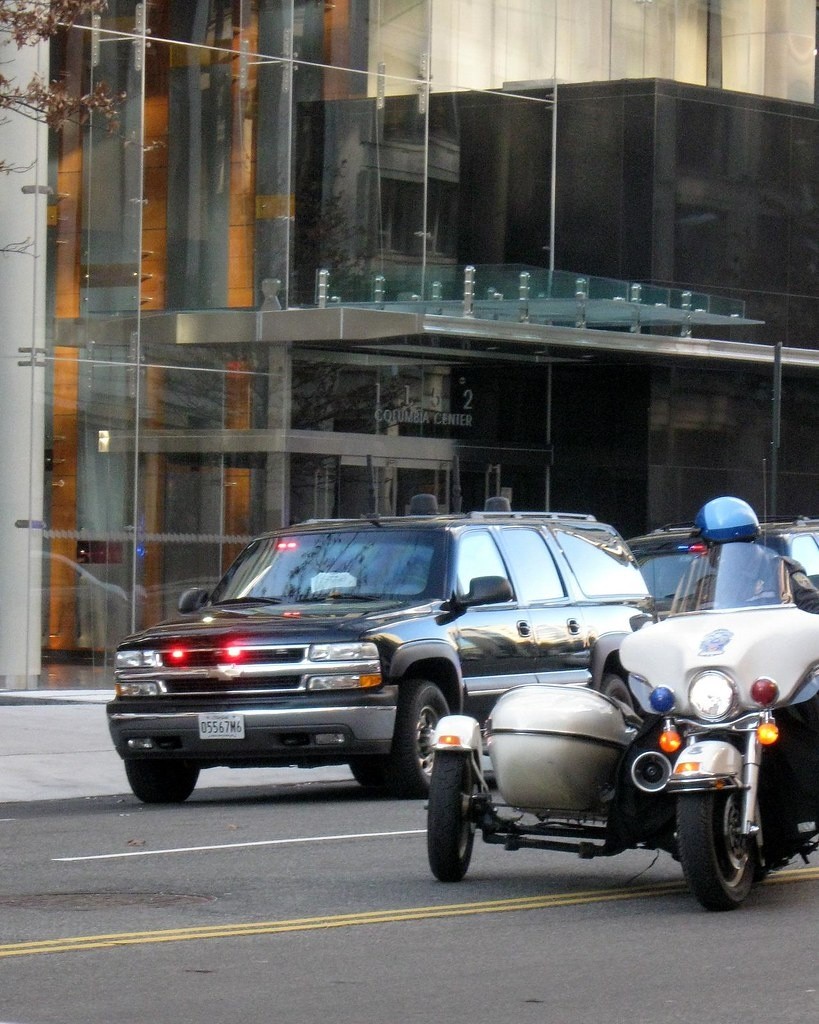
[624,514,819,612]
[106,494,662,803]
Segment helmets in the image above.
[695,496,761,543]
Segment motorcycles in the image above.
[424,541,819,912]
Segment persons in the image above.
[690,496,818,731]
